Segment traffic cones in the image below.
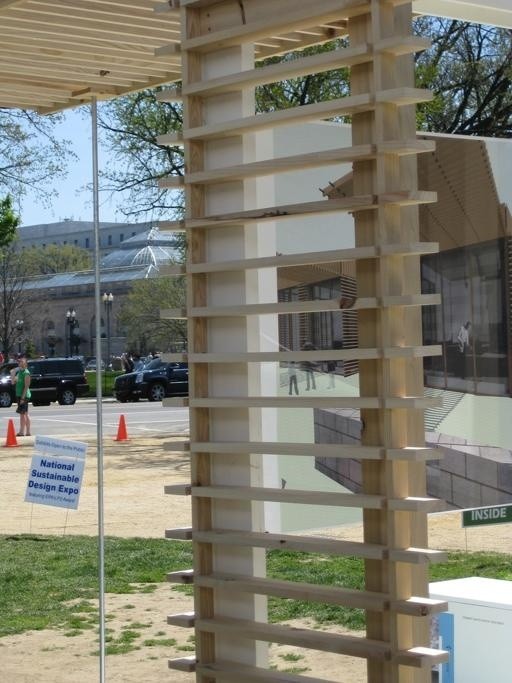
[0,418,20,448]
[111,411,131,442]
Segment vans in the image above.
[0,356,92,410]
[70,355,86,369]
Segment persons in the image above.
[327,360,337,390]
[455,319,473,379]
[9,357,33,435]
[109,348,163,373]
[303,359,317,391]
[288,359,299,395]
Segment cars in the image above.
[86,359,106,370]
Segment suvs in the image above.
[114,356,190,403]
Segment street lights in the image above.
[66,308,76,357]
[16,319,25,352]
[102,290,116,369]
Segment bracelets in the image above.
[21,397,24,400]
